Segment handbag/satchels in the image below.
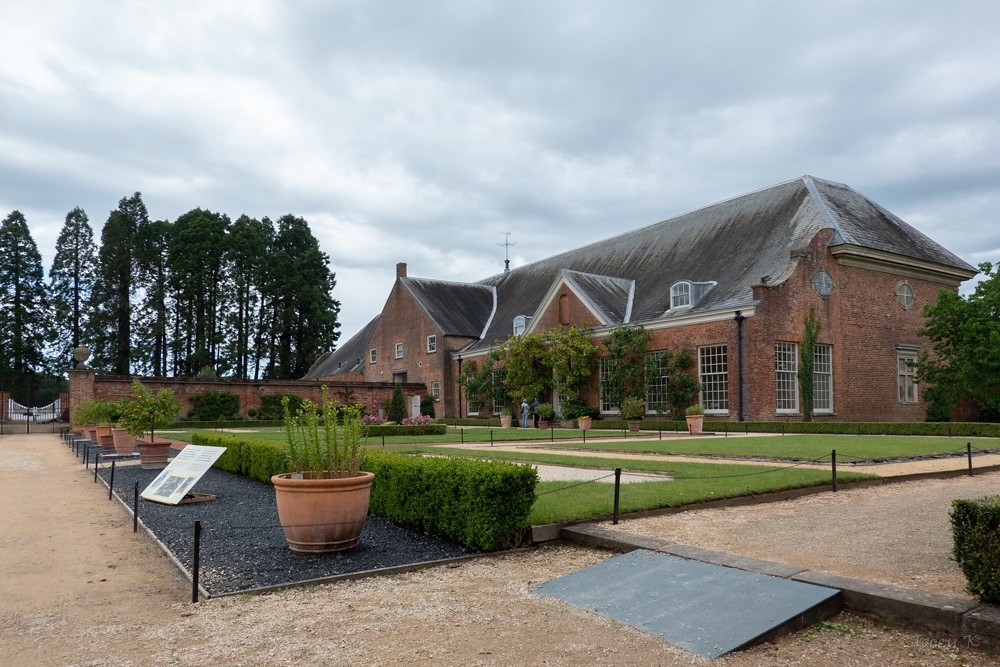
[519,404,525,415]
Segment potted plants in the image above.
[623,396,645,431]
[685,404,705,433]
[73,378,183,470]
[535,402,553,429]
[271,384,375,553]
[499,407,512,429]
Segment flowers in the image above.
[577,416,590,419]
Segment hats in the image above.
[523,398,527,403]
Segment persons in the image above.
[521,398,540,428]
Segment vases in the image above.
[577,418,592,430]
